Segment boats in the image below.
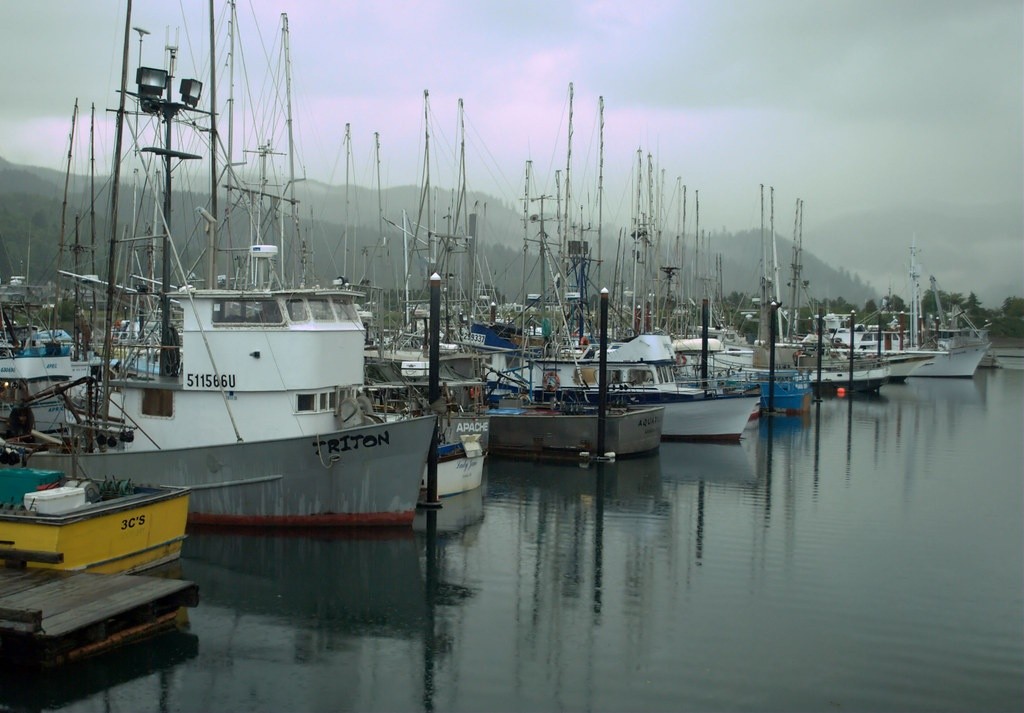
[1,469,193,578]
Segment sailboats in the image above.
[1,0,1007,541]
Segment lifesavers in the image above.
[580,336,590,352]
[676,354,687,367]
[470,386,480,398]
[544,373,560,391]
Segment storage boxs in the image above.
[0,466,65,510]
[23,486,86,515]
